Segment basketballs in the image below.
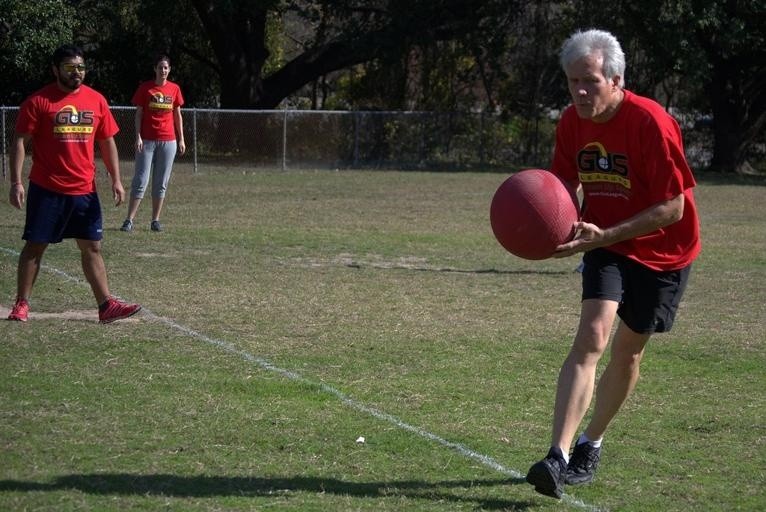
[490,168,580,260]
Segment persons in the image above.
[6,41,143,324]
[525,28,704,499]
[120,55,189,232]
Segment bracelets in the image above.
[11,181,22,188]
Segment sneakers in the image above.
[151,221,160,231]
[8,298,30,321]
[524,447,568,499]
[98,295,141,323]
[120,220,133,232]
[565,434,604,486]
